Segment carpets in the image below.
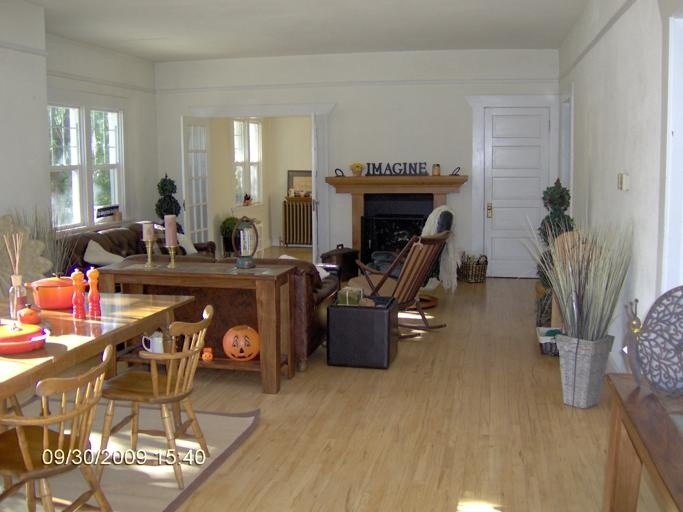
[0,391,261,512]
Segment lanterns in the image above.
[202,350,214,362]
[223,324,260,360]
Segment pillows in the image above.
[82,238,125,267]
[150,228,182,256]
[177,231,198,256]
[278,254,332,281]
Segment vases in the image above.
[555,334,614,410]
[352,171,362,177]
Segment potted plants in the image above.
[220,218,241,253]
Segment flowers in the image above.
[350,162,364,171]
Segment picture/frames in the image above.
[287,170,312,197]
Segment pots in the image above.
[24,272,91,311]
[17,304,43,324]
[0,322,51,357]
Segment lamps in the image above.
[230,216,260,269]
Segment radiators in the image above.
[277,195,313,248]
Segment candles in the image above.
[142,221,154,240]
[163,214,178,246]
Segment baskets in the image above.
[537,327,563,356]
[459,255,488,283]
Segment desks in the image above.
[96,261,298,395]
[323,292,399,370]
[606,372,683,512]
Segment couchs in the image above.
[118,253,342,372]
[56,219,218,351]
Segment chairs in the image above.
[0,344,114,512]
[93,305,215,490]
[354,235,447,339]
[349,205,454,309]
